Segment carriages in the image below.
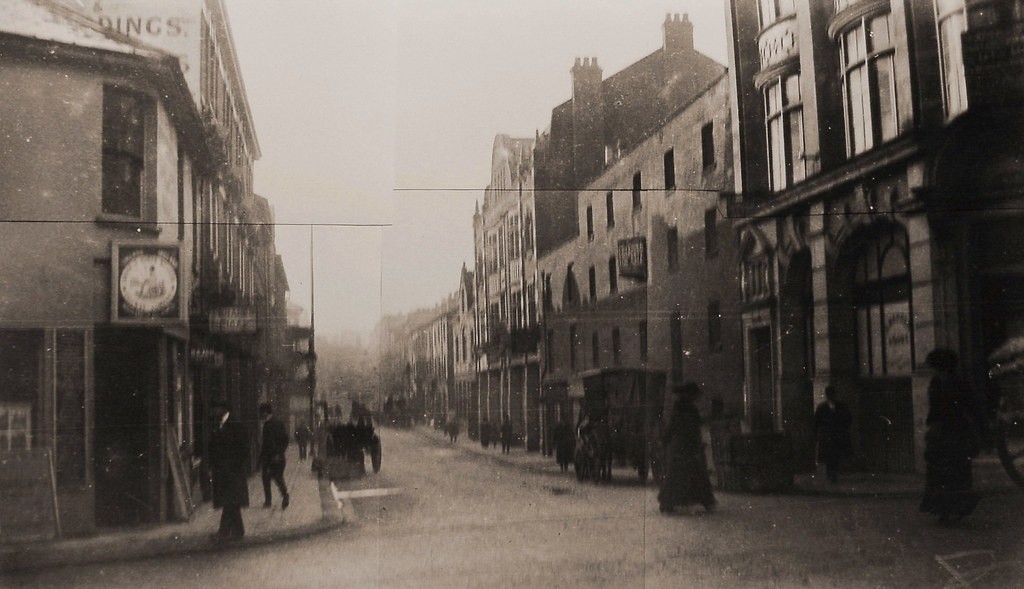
[548,369,649,486]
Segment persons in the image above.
[256,403,291,505]
[812,386,855,467]
[576,380,718,516]
[917,346,988,524]
[552,410,575,472]
[207,395,252,541]
[294,419,314,462]
[497,414,512,455]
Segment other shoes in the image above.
[209,532,230,541]
[223,528,244,544]
[263,503,270,508]
[282,494,289,510]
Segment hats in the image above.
[260,402,273,413]
[207,395,233,409]
